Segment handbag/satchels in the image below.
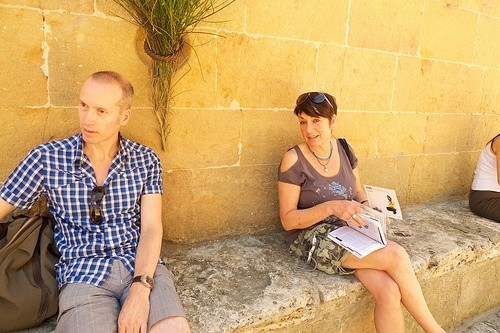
[1,189,60,333]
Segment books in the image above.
[328,205,387,259]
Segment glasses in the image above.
[297,91,334,110]
[90,186,105,224]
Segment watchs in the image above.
[130,275,154,290]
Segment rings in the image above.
[352,212,357,219]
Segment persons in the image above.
[469,134,500,223]
[277,92,446,333]
[0,71,191,333]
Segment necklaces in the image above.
[307,141,333,172]
